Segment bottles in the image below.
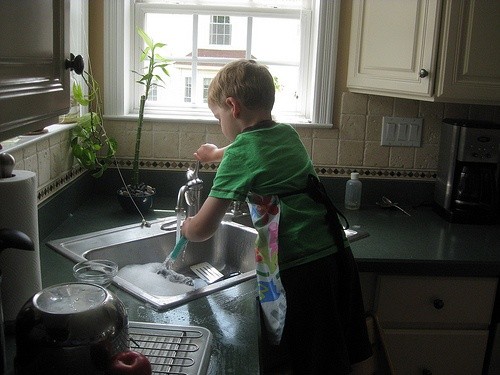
[345,173,364,210]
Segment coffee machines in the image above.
[433,117,500,224]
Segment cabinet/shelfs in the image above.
[348,270,500,375]
[346,0,500,106]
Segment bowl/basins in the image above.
[14,281,130,375]
[72,260,118,288]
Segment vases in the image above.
[58,70,80,123]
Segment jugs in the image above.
[453,165,497,208]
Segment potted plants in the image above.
[116,27,177,214]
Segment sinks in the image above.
[46,216,273,312]
[228,212,370,242]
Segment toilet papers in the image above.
[0,169,43,322]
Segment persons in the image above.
[181,59,374,375]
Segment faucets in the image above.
[174,159,204,219]
